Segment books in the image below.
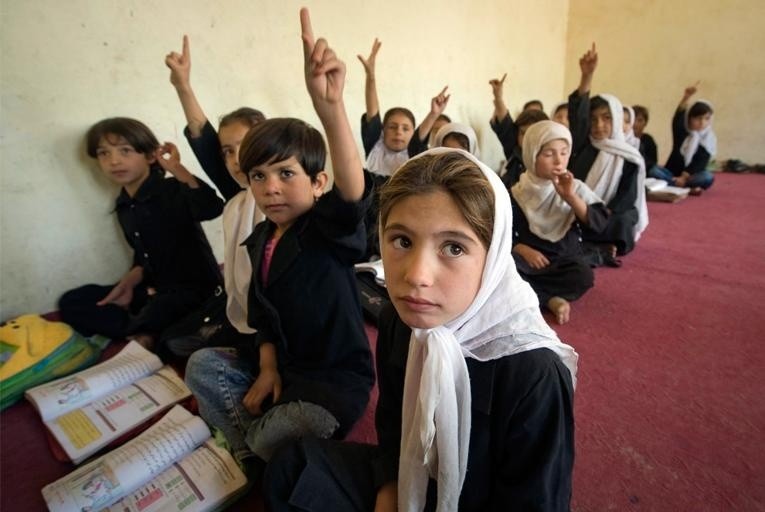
[40,402,250,512]
[24,339,194,466]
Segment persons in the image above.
[184,6,375,488]
[57,117,224,348]
[358,38,417,257]
[258,147,579,511]
[505,120,609,325]
[149,36,265,375]
[408,40,719,267]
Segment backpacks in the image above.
[0,313,112,410]
